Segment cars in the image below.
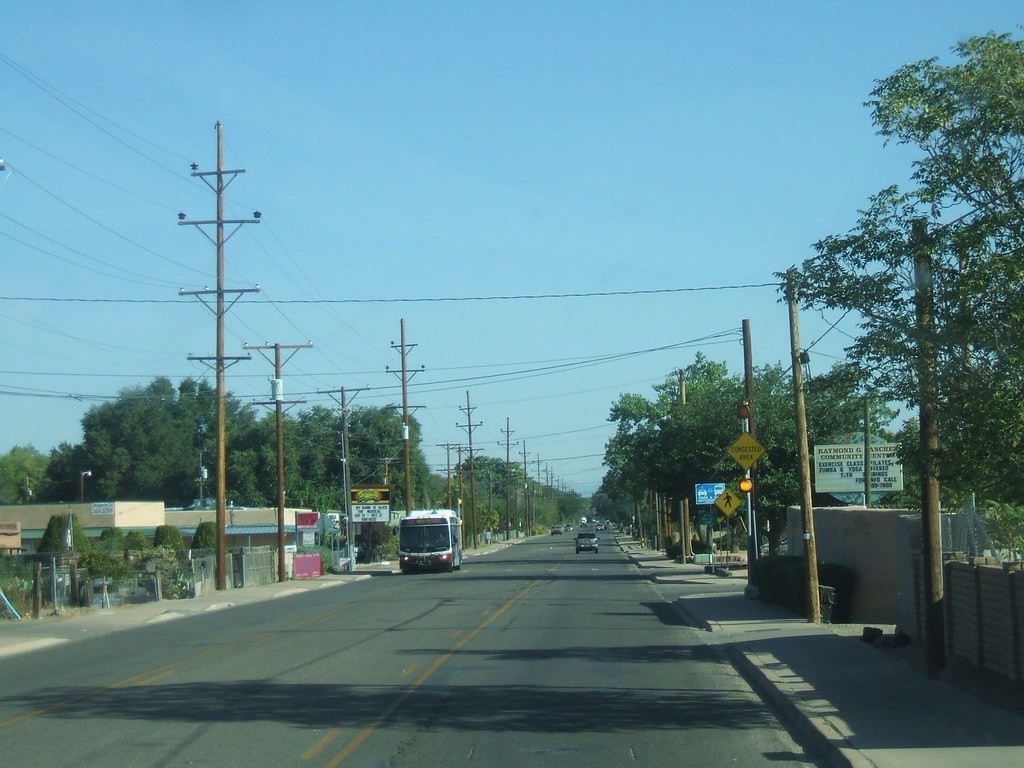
[564,524,574,531]
[591,517,600,523]
[578,517,587,528]
[596,523,604,531]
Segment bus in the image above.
[392,509,463,572]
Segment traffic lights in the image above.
[739,479,752,492]
[738,405,749,417]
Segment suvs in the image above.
[573,532,600,553]
[551,525,563,536]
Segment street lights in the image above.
[80,470,92,501]
